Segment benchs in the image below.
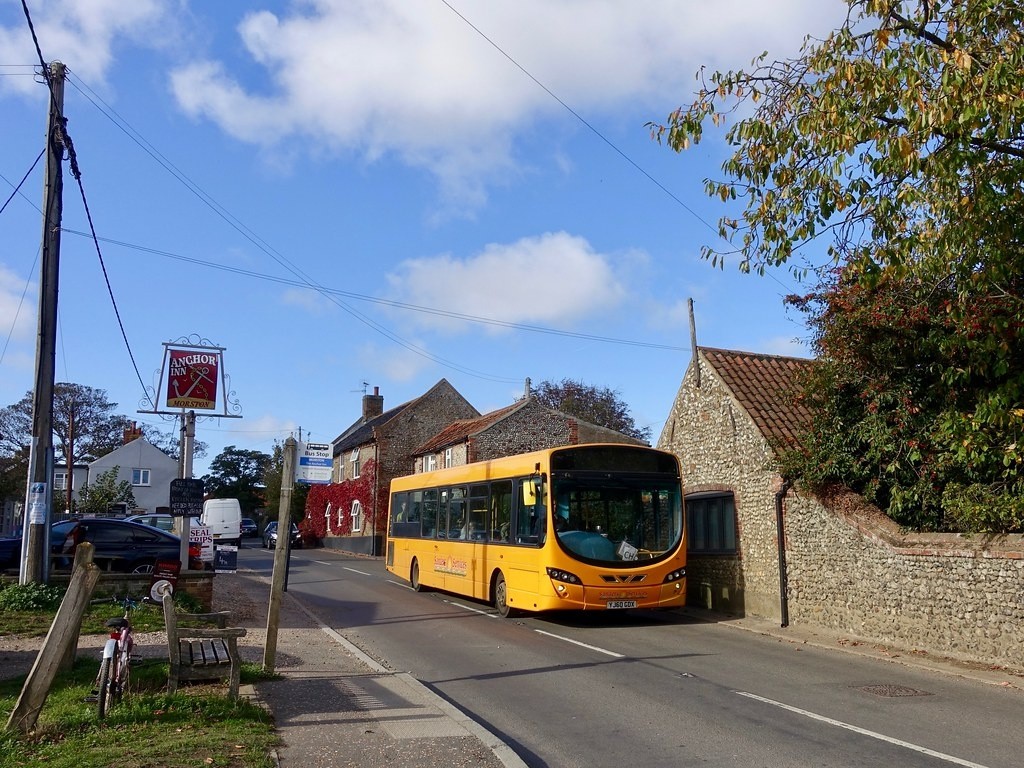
[159,587,246,701]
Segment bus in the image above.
[384,443,689,617]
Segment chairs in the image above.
[397,506,485,540]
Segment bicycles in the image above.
[84,593,154,720]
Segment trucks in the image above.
[199,498,242,548]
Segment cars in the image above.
[240,517,259,538]
[0,518,206,601]
[262,521,303,549]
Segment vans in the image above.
[124,512,201,538]
